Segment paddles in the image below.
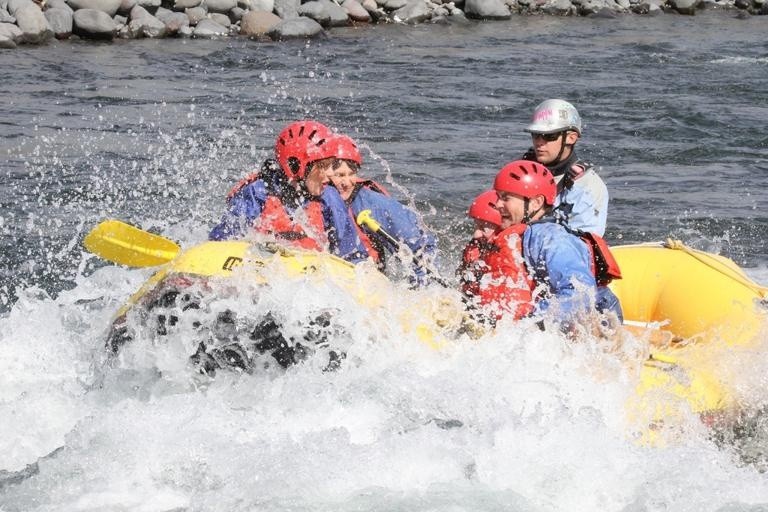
[82,220,181,267]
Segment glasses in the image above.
[531,132,561,141]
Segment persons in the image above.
[519,97,609,238]
[328,133,436,294]
[454,159,622,344]
[206,118,376,272]
[467,190,622,327]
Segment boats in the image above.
[98,239,768,448]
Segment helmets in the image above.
[335,135,361,165]
[495,160,556,205]
[469,191,502,227]
[524,99,582,137]
[276,120,337,181]
